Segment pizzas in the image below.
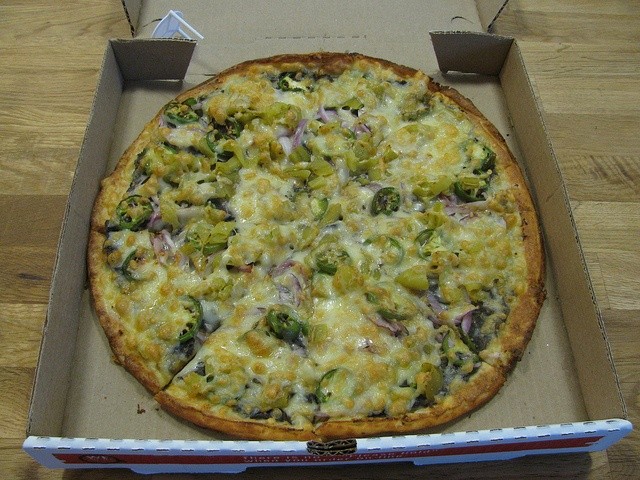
[88,50,546,441]
